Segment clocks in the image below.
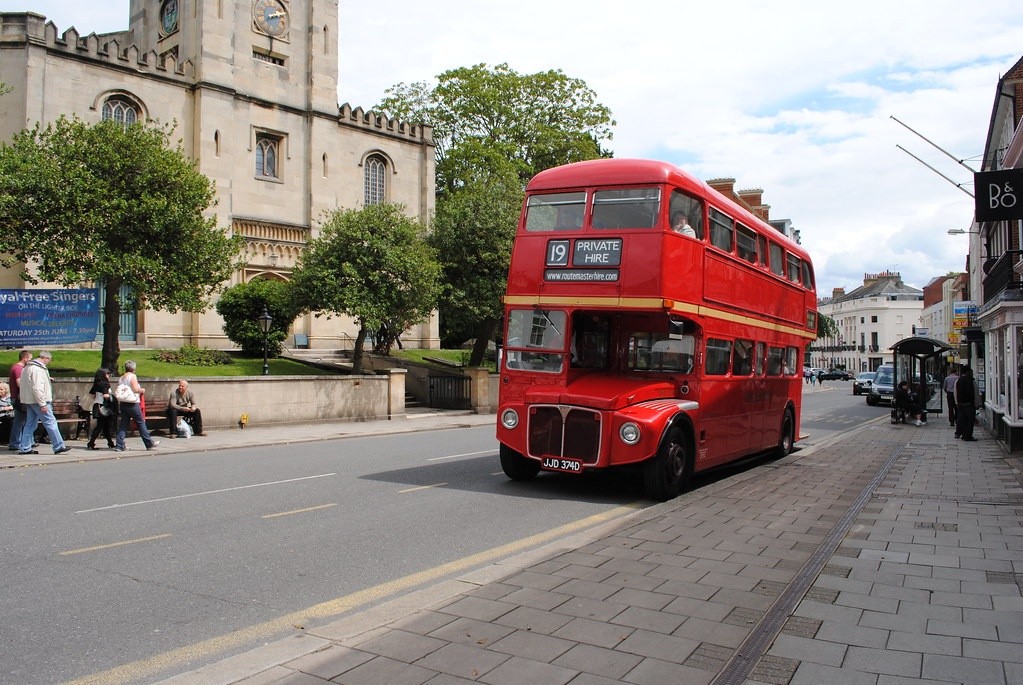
[158,0,179,38]
[252,0,290,37]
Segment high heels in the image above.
[86,442,100,450]
[107,441,116,448]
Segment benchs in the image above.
[117,397,192,435]
[0,396,93,441]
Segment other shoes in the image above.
[146,440,160,450]
[194,431,208,436]
[31,443,39,447]
[18,448,38,455]
[916,420,926,425]
[8,445,19,451]
[55,445,71,453]
[38,437,52,444]
[114,445,130,452]
[170,433,176,439]
[955,431,978,441]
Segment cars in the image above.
[802,366,850,381]
[852,372,877,395]
[915,373,937,402]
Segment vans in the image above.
[866,364,908,406]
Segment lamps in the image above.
[948,229,980,235]
[259,304,273,332]
[947,289,968,294]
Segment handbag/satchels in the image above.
[176,419,192,438]
[117,374,138,402]
[98,404,112,416]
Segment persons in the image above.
[87,369,115,450]
[954,366,980,441]
[897,382,928,426]
[548,325,577,365]
[19,351,72,454]
[115,361,161,451]
[0,383,15,444]
[805,370,822,385]
[168,380,208,439]
[9,350,39,450]
[671,210,696,238]
[944,369,959,426]
[720,347,789,376]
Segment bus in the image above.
[494,157,817,502]
